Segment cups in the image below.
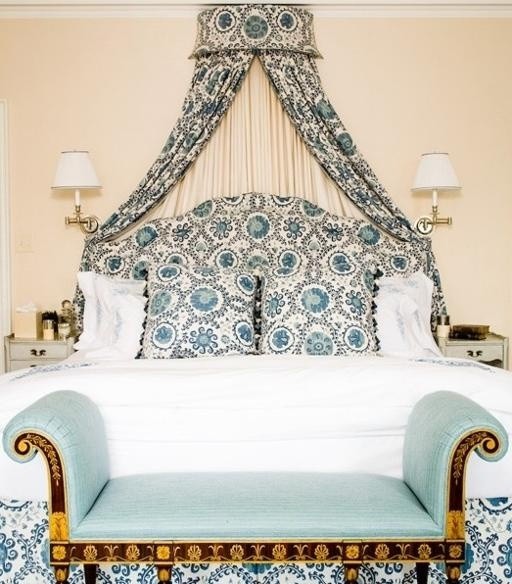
[436,315,450,335]
[41,320,55,338]
[58,323,70,335]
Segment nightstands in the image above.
[4,333,79,372]
[434,333,512,371]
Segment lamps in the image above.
[411,152,463,234]
[50,152,103,234]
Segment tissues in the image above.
[12,305,42,340]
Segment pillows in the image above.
[257,272,378,358]
[143,272,260,358]
[374,270,441,358]
[71,271,148,360]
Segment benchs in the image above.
[3,391,508,584]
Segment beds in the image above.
[2,191,512,584]
